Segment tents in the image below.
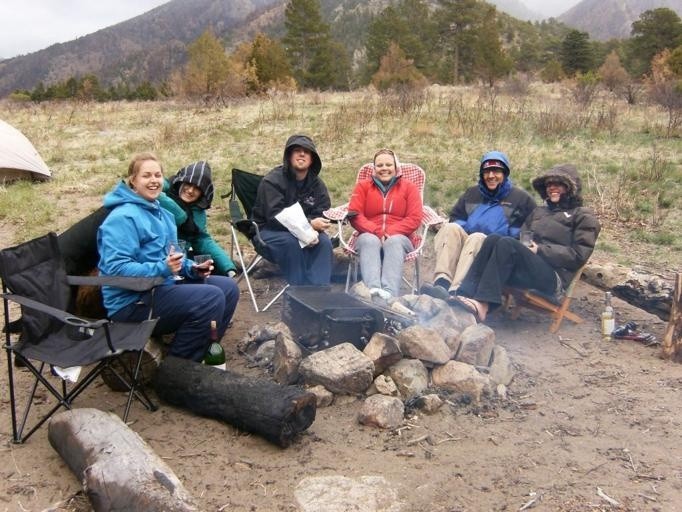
[1,120,52,183]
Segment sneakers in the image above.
[433,286,448,299]
[422,283,433,295]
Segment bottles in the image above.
[204,320,226,370]
[601,291,615,341]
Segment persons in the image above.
[251,134,335,287]
[347,146,424,299]
[95,151,240,365]
[419,150,537,298]
[444,162,602,323]
[159,158,238,278]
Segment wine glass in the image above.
[194,254,211,284]
[167,239,186,281]
[520,231,534,248]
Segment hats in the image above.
[483,161,505,169]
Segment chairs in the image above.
[0,231,164,444]
[220,169,340,312]
[323,163,443,294]
[502,254,590,334]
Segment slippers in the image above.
[615,322,650,340]
[448,294,481,324]
